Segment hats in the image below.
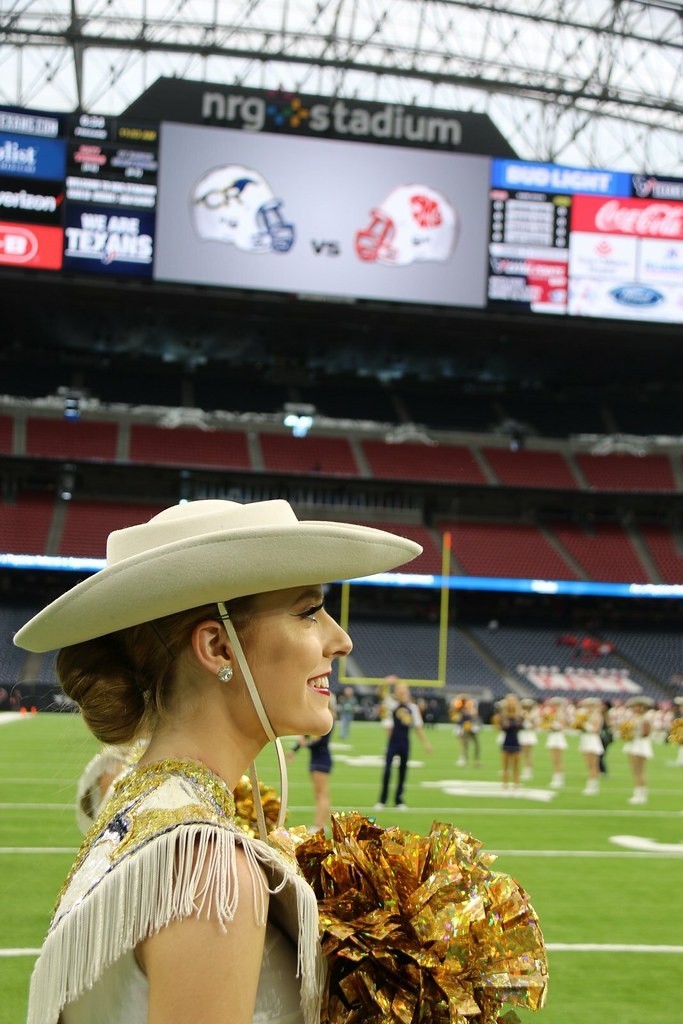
[12,498,423,654]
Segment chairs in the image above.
[0,490,683,586]
[0,408,683,493]
[0,609,682,702]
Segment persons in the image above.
[495,693,570,789]
[286,692,336,834]
[621,696,654,804]
[338,687,358,739]
[74,692,153,835]
[452,692,482,767]
[13,499,426,1023]
[670,697,683,767]
[569,696,613,793]
[375,674,431,811]
